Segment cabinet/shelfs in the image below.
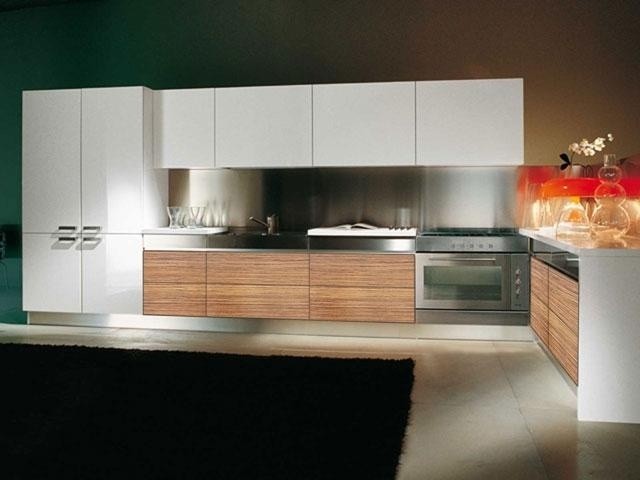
[214,77,525,171]
[152,87,215,170]
[21,234,145,315]
[19,84,167,234]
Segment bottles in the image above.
[523,182,554,231]
[590,154,630,240]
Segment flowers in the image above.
[558,131,615,177]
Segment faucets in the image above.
[248,215,280,235]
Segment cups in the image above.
[166,205,206,229]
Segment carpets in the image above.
[0,341,414,478]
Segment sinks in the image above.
[207,233,308,250]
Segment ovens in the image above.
[415,252,530,310]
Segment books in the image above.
[333,221,375,230]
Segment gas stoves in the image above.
[416,231,531,252]
